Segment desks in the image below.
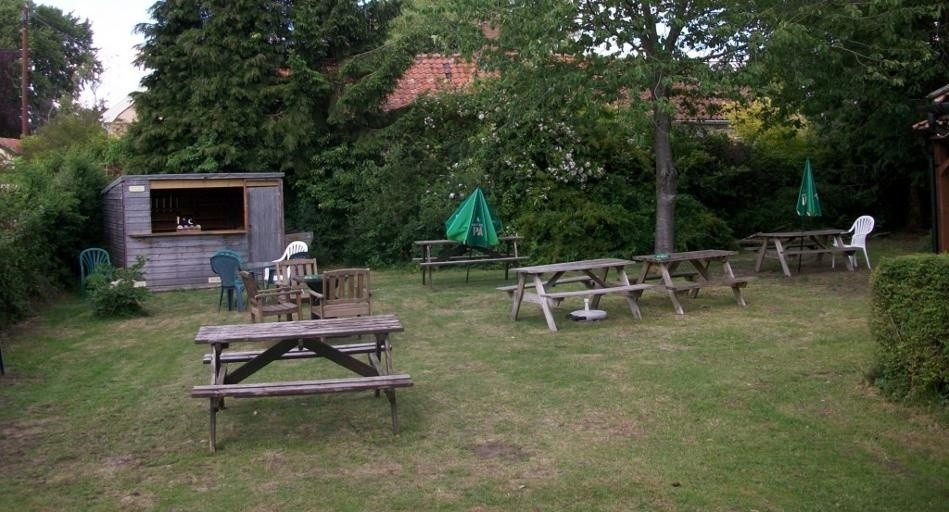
[754,229,854,279]
[414,237,524,290]
[632,249,746,314]
[194,314,404,452]
[508,258,642,332]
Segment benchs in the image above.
[412,254,531,292]
[745,243,857,279]
[496,272,760,332]
[191,342,414,452]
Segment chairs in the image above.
[832,215,875,269]
[80,248,112,295]
[210,241,372,343]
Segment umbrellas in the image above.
[795,154,823,274]
[444,184,500,286]
